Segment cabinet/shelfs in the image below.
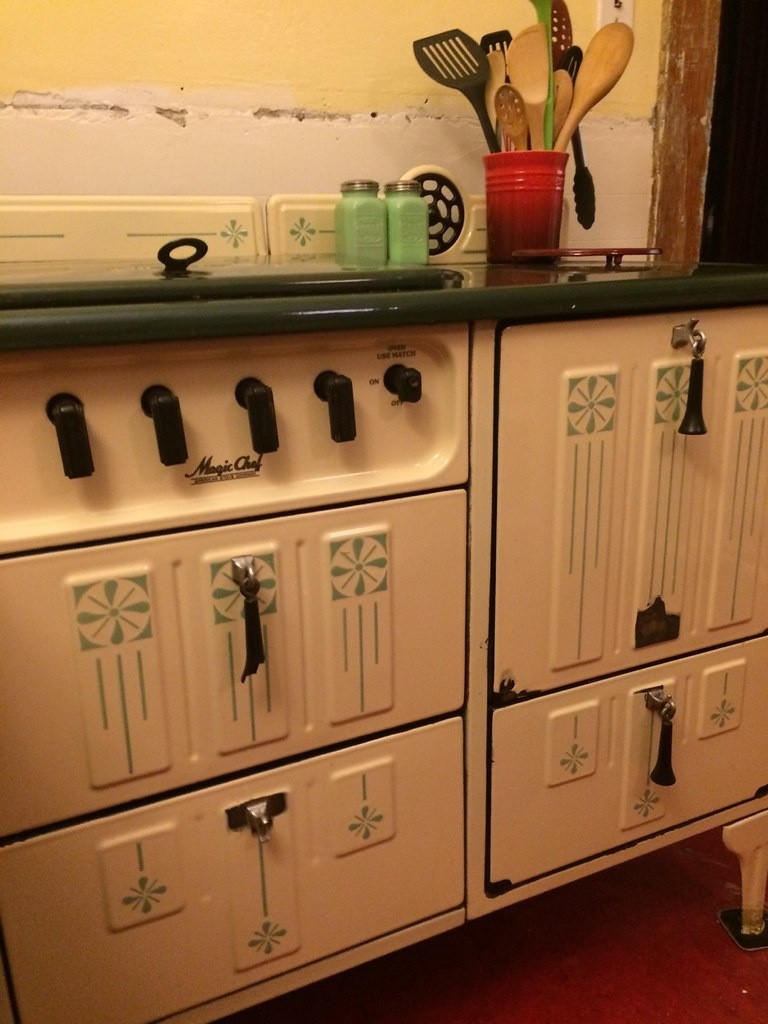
[0,309,768,1024]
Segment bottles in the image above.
[383,180,430,268]
[334,178,388,268]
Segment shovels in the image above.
[412,28,499,152]
[482,22,550,150]
[551,0,574,72]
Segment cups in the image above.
[481,150,570,264]
